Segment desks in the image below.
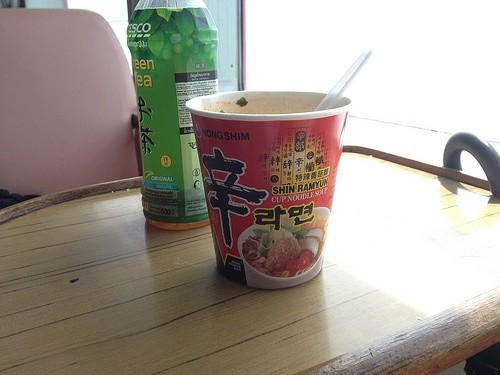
[0,138,500,373]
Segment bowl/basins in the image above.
[237,207,331,289]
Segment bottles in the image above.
[186,89,351,290]
[126,0,219,230]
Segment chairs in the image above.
[0,8,143,195]
